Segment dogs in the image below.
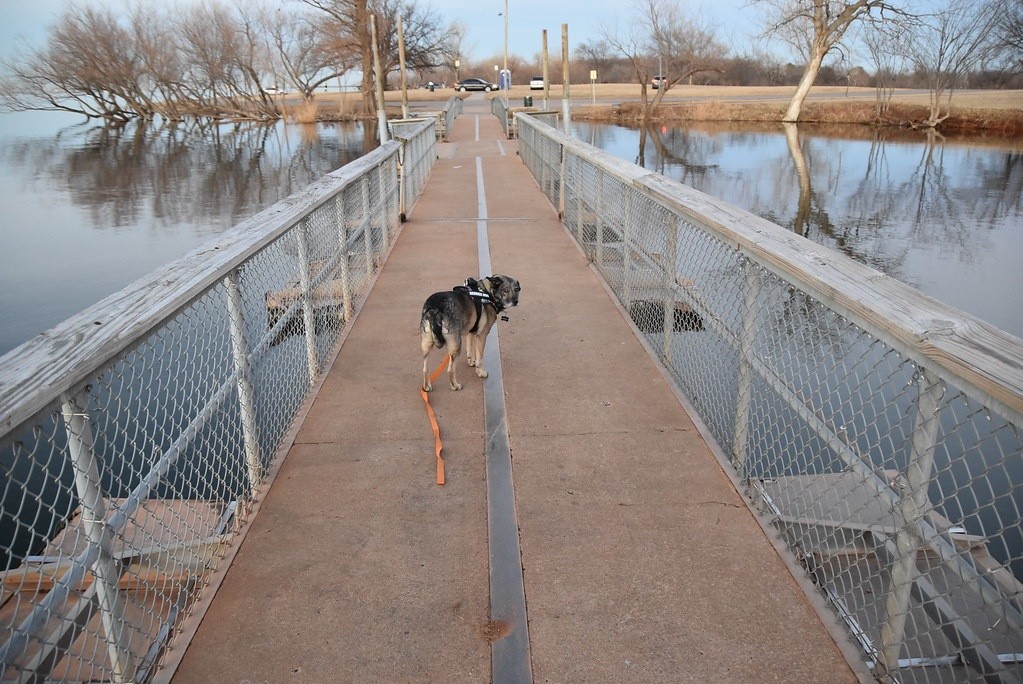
[420,274,521,393]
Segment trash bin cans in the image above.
[524,96,532,107]
[430,85,434,92]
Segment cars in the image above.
[263,86,288,96]
[530,76,551,90]
[455,78,500,93]
[652,76,669,90]
